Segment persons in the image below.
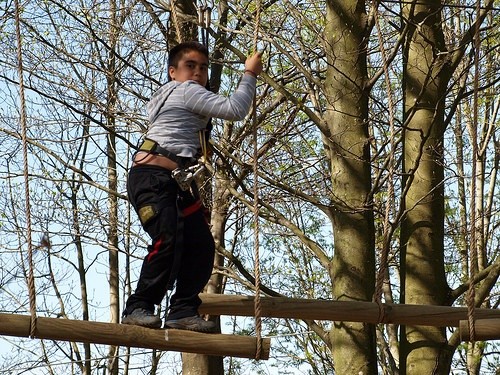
[120,42,264,332]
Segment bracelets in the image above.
[244,69,258,79]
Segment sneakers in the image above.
[164,316,216,332]
[122,308,162,329]
